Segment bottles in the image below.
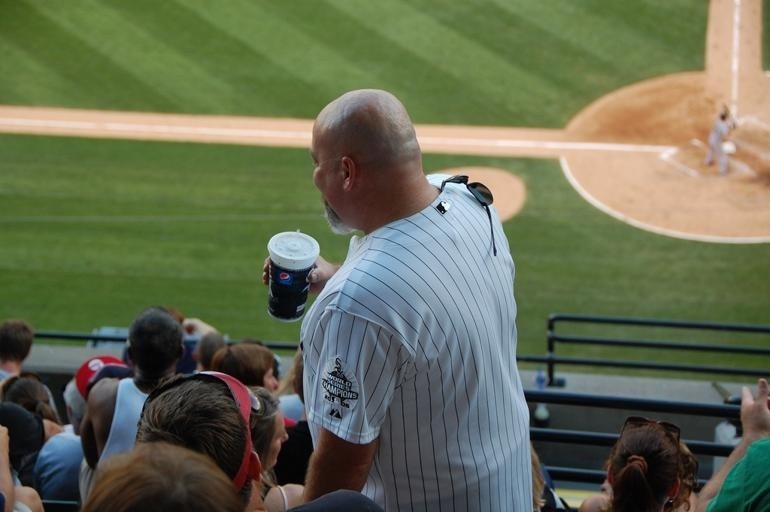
[535,366,545,389]
[533,402,551,428]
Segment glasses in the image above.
[441,174,497,255]
[618,417,680,453]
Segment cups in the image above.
[267,230,321,324]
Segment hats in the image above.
[144,372,262,492]
[76,357,127,396]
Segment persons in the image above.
[664,442,700,511]
[258,85,537,512]
[573,416,685,512]
[703,102,736,175]
[693,378,769,511]
[709,379,744,480]
[1,303,389,511]
